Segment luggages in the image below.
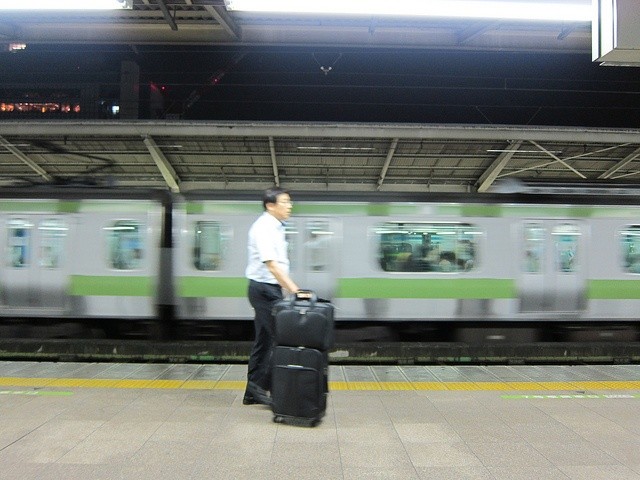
[266,289,329,427]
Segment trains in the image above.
[1,192,639,343]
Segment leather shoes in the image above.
[249,383,270,402]
[243,393,262,406]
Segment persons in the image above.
[242,186,310,406]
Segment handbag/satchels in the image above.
[271,289,334,351]
